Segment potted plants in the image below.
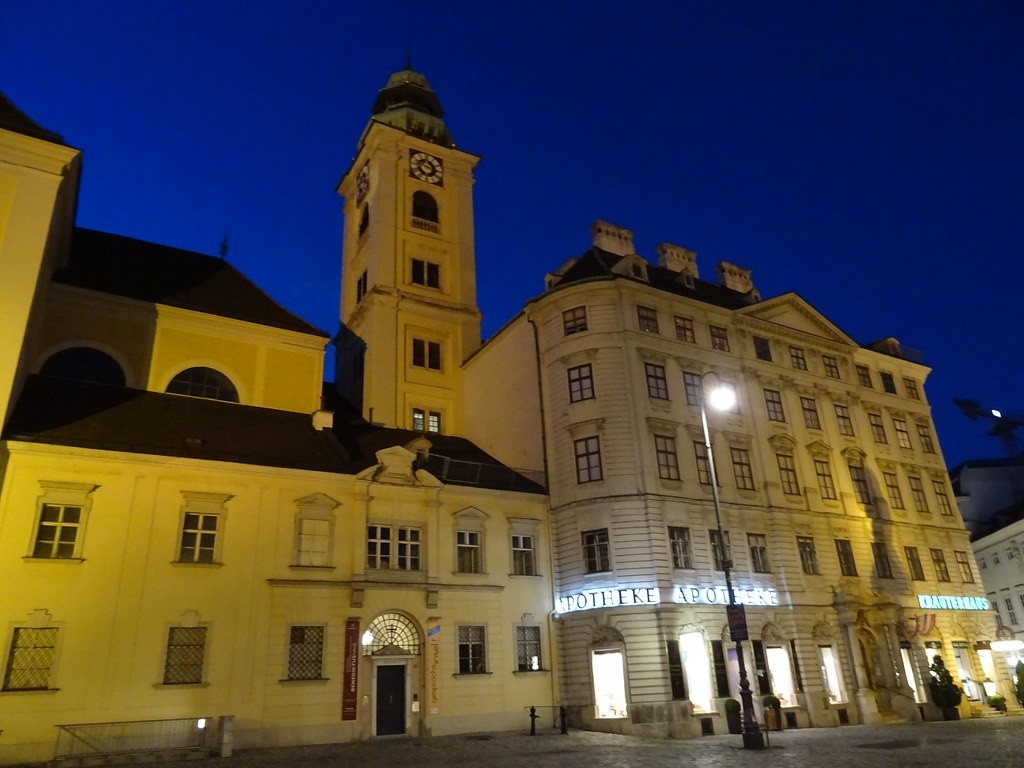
[725,698,742,733]
[928,655,962,720]
[763,696,781,730]
[986,695,1007,713]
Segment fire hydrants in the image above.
[559,704,568,735]
[530,706,540,736]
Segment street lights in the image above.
[699,369,767,752]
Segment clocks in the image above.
[410,152,443,183]
